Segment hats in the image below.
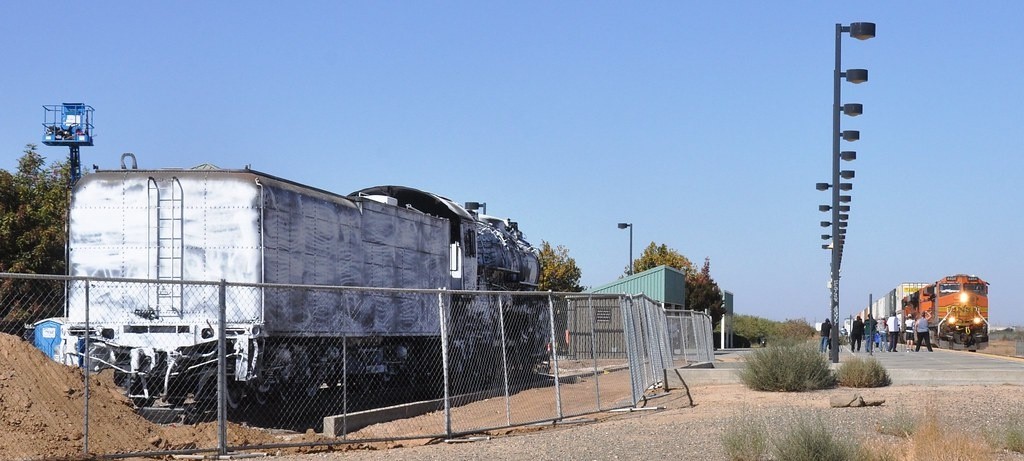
[908,314,911,317]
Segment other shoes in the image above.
[888,349,891,352]
[893,350,897,352]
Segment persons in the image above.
[851,314,889,352]
[914,311,933,352]
[820,318,831,352]
[886,312,900,352]
[905,314,915,352]
[840,326,845,336]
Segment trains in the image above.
[844,273,989,352]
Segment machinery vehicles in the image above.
[65,153,551,430]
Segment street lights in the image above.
[816,21,876,362]
[618,223,632,276]
